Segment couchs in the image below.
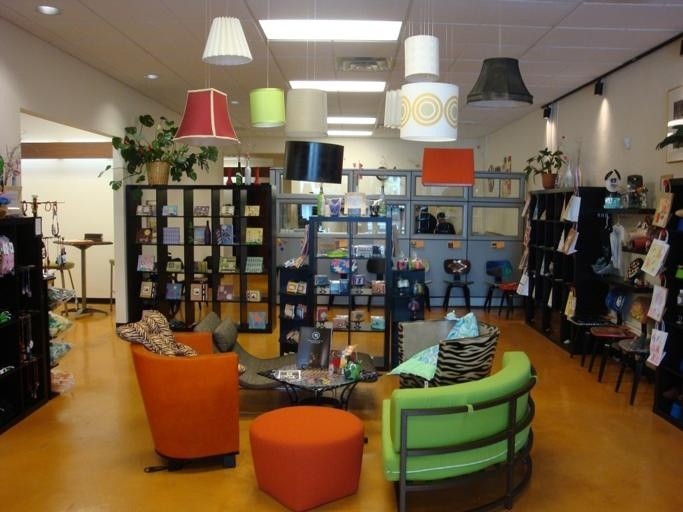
[381,350,538,512]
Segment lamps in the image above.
[541,32,683,119]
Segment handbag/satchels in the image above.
[641,230,670,276]
[647,319,669,366]
[652,183,674,227]
[516,270,529,296]
[562,189,581,222]
[648,275,669,322]
[518,246,529,270]
[523,220,532,246]
[562,222,580,255]
[564,288,578,316]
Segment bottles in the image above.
[562,159,573,186]
[255,167,261,184]
[203,220,210,244]
[343,359,361,379]
[573,164,582,185]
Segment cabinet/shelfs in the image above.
[0,217,60,435]
[309,217,392,371]
[391,266,425,370]
[652,178,683,432]
[526,186,611,350]
[598,207,655,294]
[125,183,276,334]
[278,266,315,356]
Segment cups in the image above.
[245,159,252,187]
[346,195,363,218]
[327,196,342,218]
[368,197,380,218]
[225,165,233,187]
[235,160,243,186]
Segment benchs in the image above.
[398,318,501,388]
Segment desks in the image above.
[53,240,113,315]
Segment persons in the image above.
[435,212,456,233]
[414,205,437,234]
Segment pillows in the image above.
[386,312,479,381]
[213,316,237,352]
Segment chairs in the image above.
[423,260,523,319]
[130,332,239,471]
[193,312,377,415]
[580,291,654,405]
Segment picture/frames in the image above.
[4,185,22,215]
[665,85,683,163]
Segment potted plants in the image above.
[524,148,568,189]
[97,115,218,190]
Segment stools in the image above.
[109,259,114,311]
[249,406,365,512]
[43,263,79,316]
